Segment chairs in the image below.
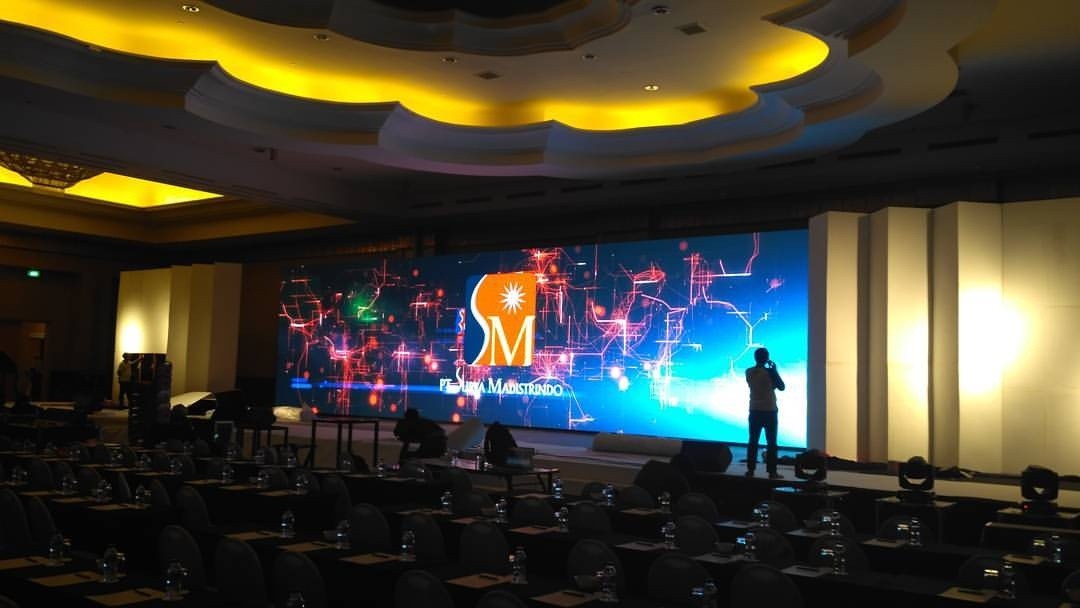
[0,437,857,608]
[879,515,932,546]
[957,556,1030,591]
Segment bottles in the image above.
[553,476,569,529]
[450,448,485,472]
[442,491,451,511]
[601,560,617,601]
[513,545,528,584]
[22,436,388,496]
[62,535,183,601]
[703,579,717,608]
[496,491,509,520]
[833,545,847,574]
[831,512,839,535]
[761,503,769,525]
[999,562,1016,599]
[745,533,756,559]
[662,524,674,548]
[661,488,672,512]
[910,516,920,545]
[1051,536,1063,563]
[607,483,615,505]
[282,515,416,562]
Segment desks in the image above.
[0,495,1063,608]
[0,403,426,522]
[414,456,560,494]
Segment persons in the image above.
[746,347,785,478]
[118,353,142,409]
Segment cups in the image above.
[736,537,745,559]
[895,521,910,544]
[692,587,703,608]
[1033,536,1045,561]
[983,569,999,600]
